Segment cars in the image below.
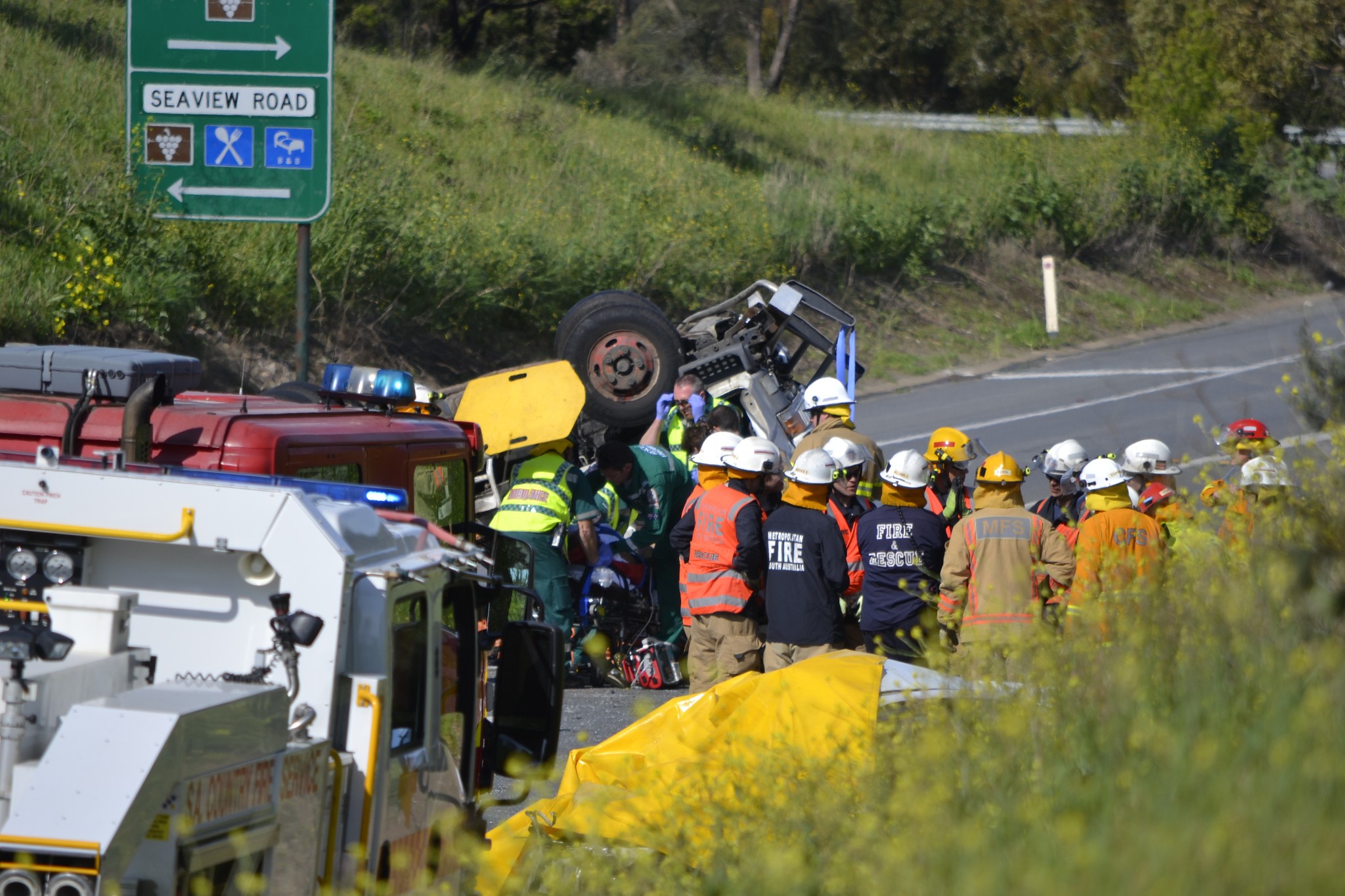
[399,277,868,532]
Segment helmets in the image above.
[1214,420,1280,449]
[784,447,848,490]
[974,451,1025,487]
[802,377,858,412]
[1121,439,1182,474]
[720,436,785,492]
[690,431,744,467]
[879,448,934,488]
[923,427,990,469]
[1034,439,1089,481]
[1240,455,1294,486]
[1137,481,1174,512]
[1060,457,1133,495]
[822,436,876,468]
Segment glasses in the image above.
[673,401,690,407]
[686,451,696,460]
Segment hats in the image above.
[597,441,633,469]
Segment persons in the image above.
[821,436,877,646]
[761,448,851,673]
[923,427,973,538]
[1121,438,1197,538]
[1066,458,1170,640]
[414,462,447,525]
[681,405,740,484]
[938,451,1076,683]
[1029,439,1095,625]
[789,377,885,500]
[490,438,612,673]
[857,449,952,664]
[584,440,694,654]
[669,431,785,694]
[1201,419,1295,558]
[639,374,739,470]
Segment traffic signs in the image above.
[121,1,336,226]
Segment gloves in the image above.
[656,394,673,420]
[588,556,610,567]
[956,477,969,518]
[597,544,613,568]
[939,626,959,654]
[838,597,848,617]
[688,394,705,424]
[942,477,960,522]
[851,594,864,622]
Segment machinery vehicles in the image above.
[0,340,564,896]
